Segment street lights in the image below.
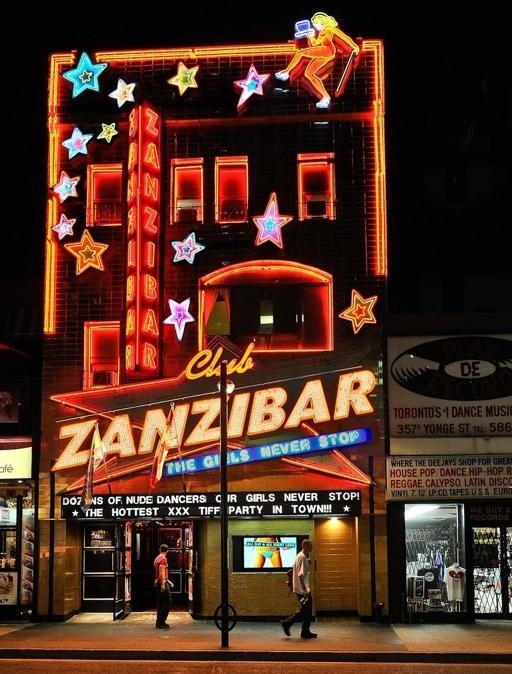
[216,360,236,649]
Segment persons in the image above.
[153,544,174,629]
[279,539,319,638]
[253,537,282,568]
[275,11,360,109]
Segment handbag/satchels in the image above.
[285,569,293,592]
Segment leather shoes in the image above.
[301,632,317,638]
[156,621,169,629]
[280,620,290,636]
